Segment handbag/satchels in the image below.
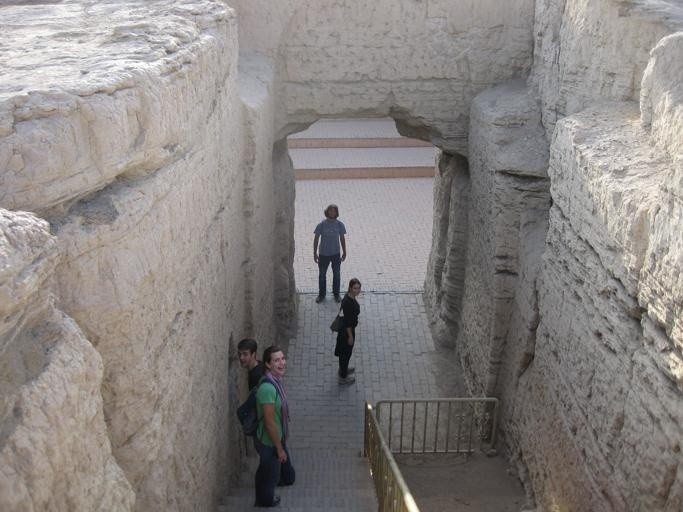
[331,315,344,331]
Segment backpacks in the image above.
[237,378,278,435]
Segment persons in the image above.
[250,345,288,507]
[236,338,295,488]
[332,278,362,385]
[312,204,347,304]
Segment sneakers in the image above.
[347,368,355,374]
[255,495,281,506]
[339,376,356,386]
[334,293,341,303]
[316,294,324,303]
